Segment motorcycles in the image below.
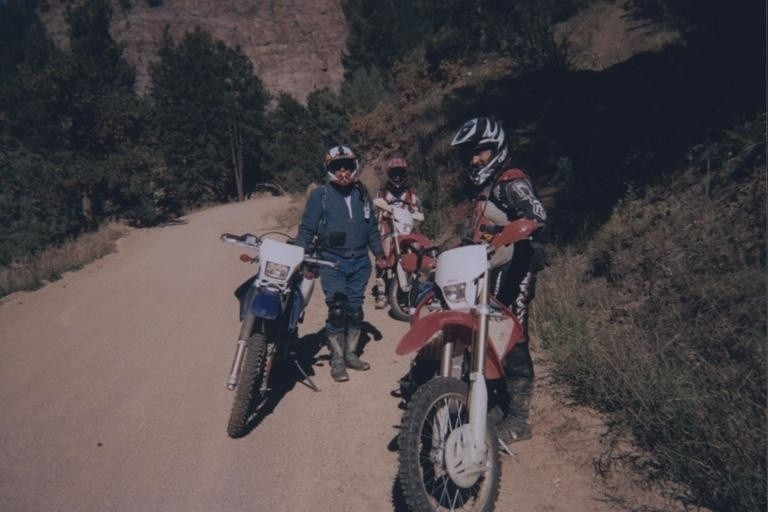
[219,229,340,438]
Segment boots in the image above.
[326,329,370,382]
[489,381,533,445]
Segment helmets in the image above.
[384,158,409,195]
[323,146,360,186]
[449,118,509,186]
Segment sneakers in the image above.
[374,294,389,310]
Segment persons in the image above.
[375,157,425,310]
[296,144,387,381]
[451,117,547,444]
